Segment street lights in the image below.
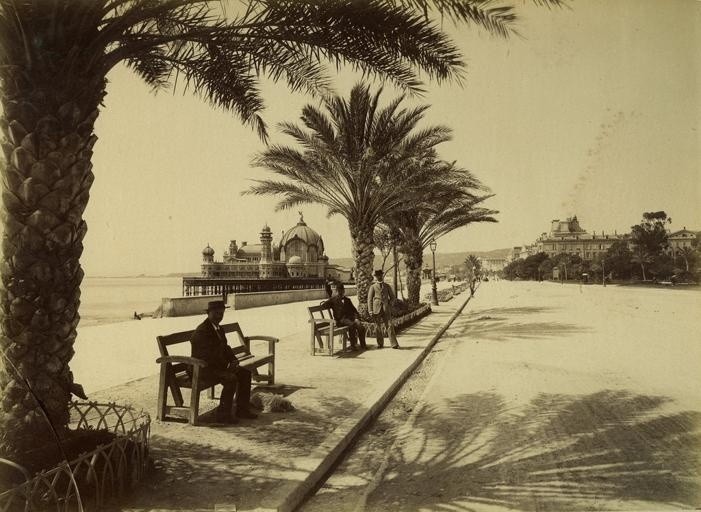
[601,258,606,288]
[429,235,440,306]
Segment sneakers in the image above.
[349,342,400,350]
[217,408,257,423]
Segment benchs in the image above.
[306,303,360,359]
[154,320,280,425]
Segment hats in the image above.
[335,284,346,289]
[204,301,230,311]
[374,270,384,276]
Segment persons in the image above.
[368,270,400,348]
[187,300,257,424]
[321,283,367,351]
[325,280,332,297]
